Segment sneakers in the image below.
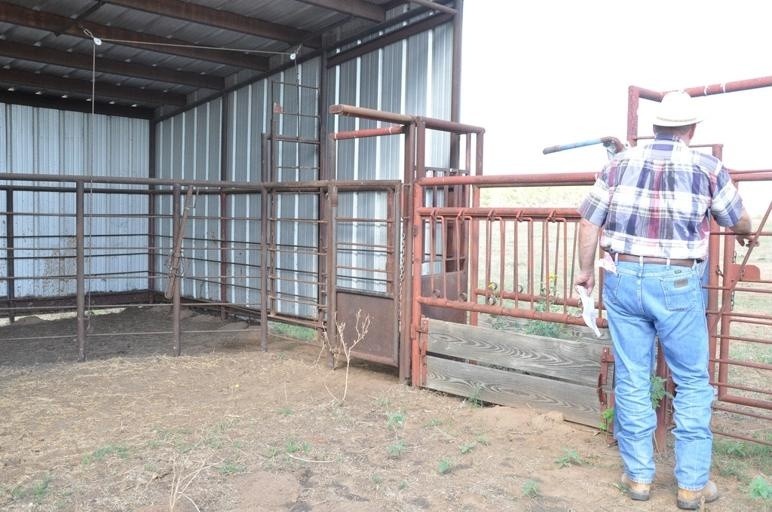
[677,480,718,510]
[621,473,651,501]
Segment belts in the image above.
[611,254,694,267]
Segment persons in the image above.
[573,91,758,511]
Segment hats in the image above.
[653,92,704,128]
